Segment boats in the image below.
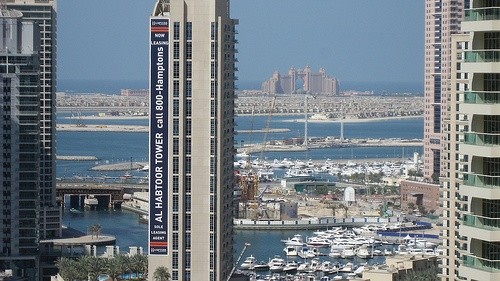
[69,208,80,214]
[233,151,424,183]
[241,222,439,281]
[138,214,148,224]
